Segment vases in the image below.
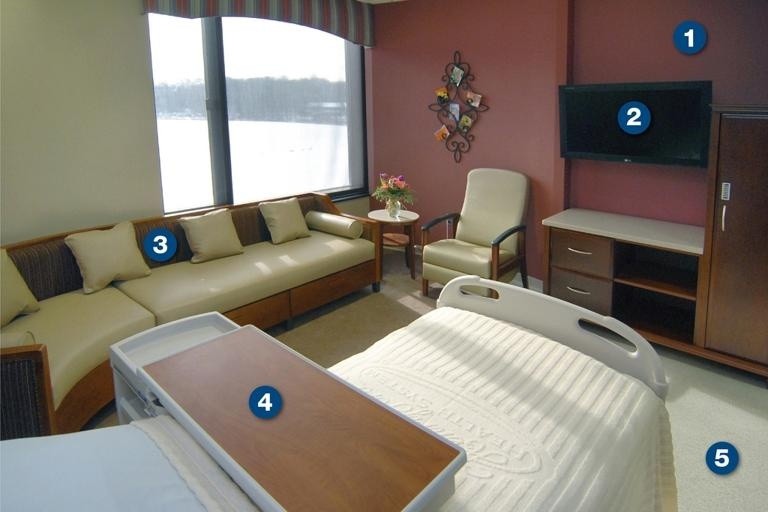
[388,198,400,216]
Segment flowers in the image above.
[371,170,414,210]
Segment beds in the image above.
[0,272,679,512]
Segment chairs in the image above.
[420,167,531,299]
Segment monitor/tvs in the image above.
[558,80,712,169]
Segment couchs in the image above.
[0,190,384,440]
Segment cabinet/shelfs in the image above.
[693,103,768,378]
[540,205,705,350]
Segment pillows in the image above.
[0,247,41,328]
[0,330,37,349]
[257,196,311,245]
[178,207,246,263]
[64,219,152,295]
[305,210,364,239]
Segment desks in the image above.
[107,310,469,512]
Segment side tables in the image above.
[367,208,420,282]
